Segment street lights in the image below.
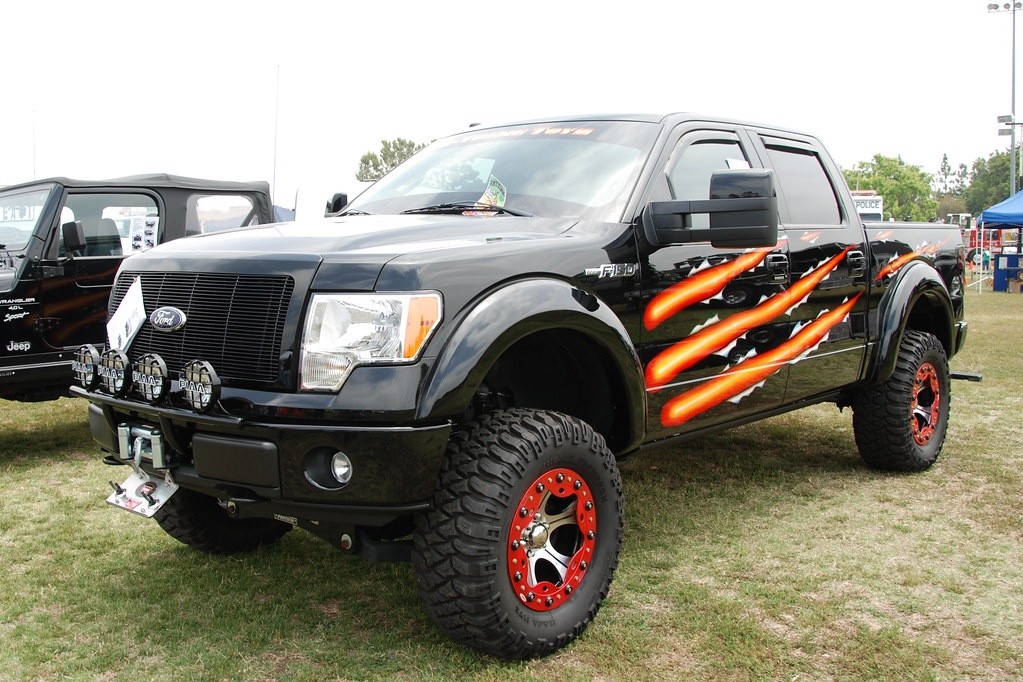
[988,0,1022,196]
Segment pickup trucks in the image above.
[68,114,985,658]
[59,235,133,257]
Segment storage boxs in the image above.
[1008,281,1023,294]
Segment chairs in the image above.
[82,218,122,256]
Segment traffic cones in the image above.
[968,260,975,270]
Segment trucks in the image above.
[943,212,1023,265]
[851,190,895,223]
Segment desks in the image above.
[1004,267,1023,293]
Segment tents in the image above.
[975,189,1023,294]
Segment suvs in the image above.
[0,173,277,402]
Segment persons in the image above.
[982,245,990,275]
